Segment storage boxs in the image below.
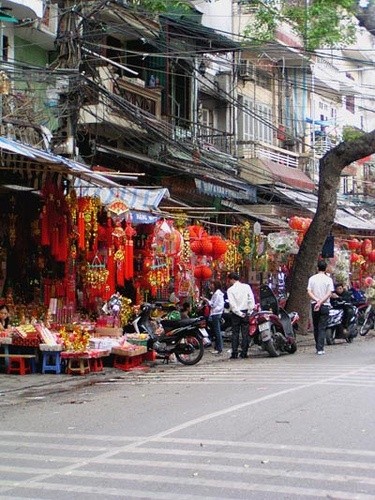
[0,324,145,352]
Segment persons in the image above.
[226,272,255,359]
[306,260,334,354]
[330,283,354,334]
[180,302,192,319]
[200,279,225,354]
[0,305,10,331]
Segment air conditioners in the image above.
[239,57,255,77]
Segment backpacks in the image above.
[350,288,367,303]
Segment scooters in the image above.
[129,301,204,366]
[327,299,359,344]
[254,284,295,357]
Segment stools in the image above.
[8,351,147,376]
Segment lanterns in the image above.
[289,216,313,236]
[190,238,212,255]
[212,237,226,259]
[194,266,213,281]
[348,237,375,270]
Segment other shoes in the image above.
[230,355,237,359]
[317,351,326,355]
[209,349,222,354]
[242,354,249,358]
